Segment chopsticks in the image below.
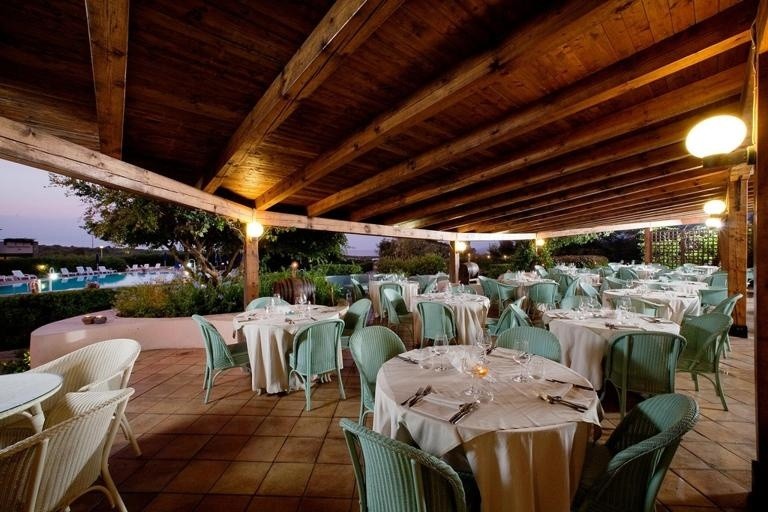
[544,376,605,392]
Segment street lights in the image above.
[99,245,104,261]
[187,257,196,273]
[48,267,57,291]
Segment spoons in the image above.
[539,391,591,414]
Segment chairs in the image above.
[415,301,459,348]
[347,325,408,445]
[0,388,135,512]
[682,313,734,412]
[192,314,254,403]
[586,392,699,512]
[245,296,289,309]
[494,326,562,364]
[336,417,467,512]
[287,319,347,411]
[598,330,688,416]
[344,261,743,328]
[712,293,743,360]
[24,336,143,459]
[0,263,161,287]
[340,297,372,351]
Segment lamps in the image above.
[685,114,756,171]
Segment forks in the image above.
[398,384,431,408]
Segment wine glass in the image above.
[512,262,576,283]
[461,352,481,397]
[431,332,448,375]
[510,337,530,384]
[578,293,631,322]
[476,327,494,363]
[266,292,307,319]
[623,277,669,295]
[684,260,711,275]
[381,272,465,303]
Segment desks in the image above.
[542,305,681,390]
[406,292,491,347]
[233,305,345,393]
[372,340,598,510]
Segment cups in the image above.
[526,356,544,381]
[583,259,652,278]
[471,377,494,402]
